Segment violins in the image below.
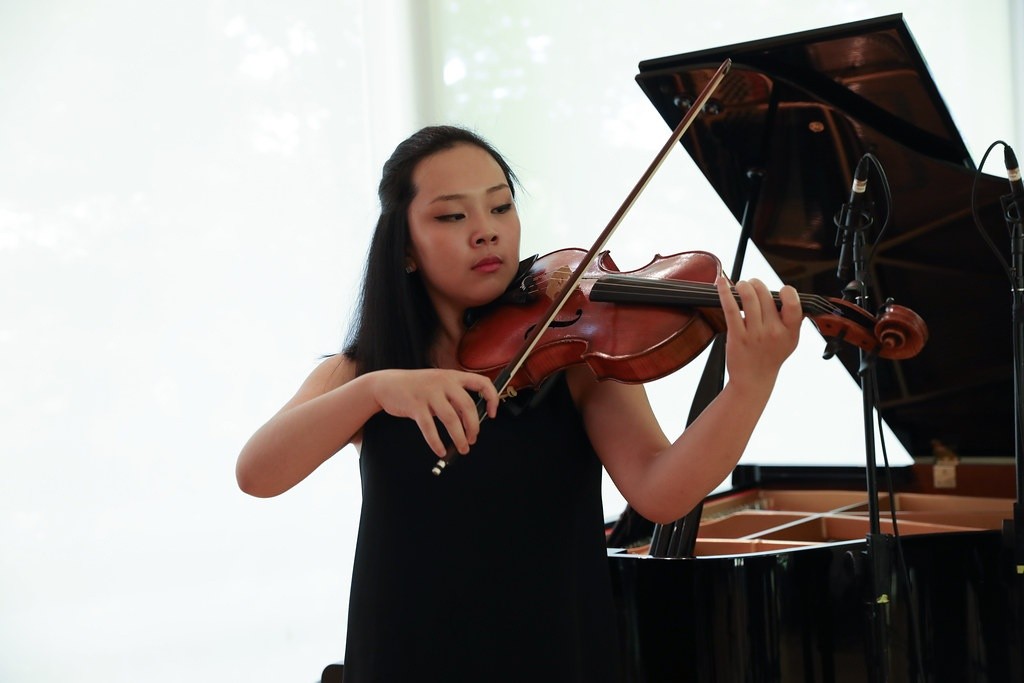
[443,247,930,402]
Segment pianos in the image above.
[291,7,1024,683]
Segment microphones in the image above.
[837,157,871,281]
[1003,146,1024,231]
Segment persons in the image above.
[237,125,802,683]
